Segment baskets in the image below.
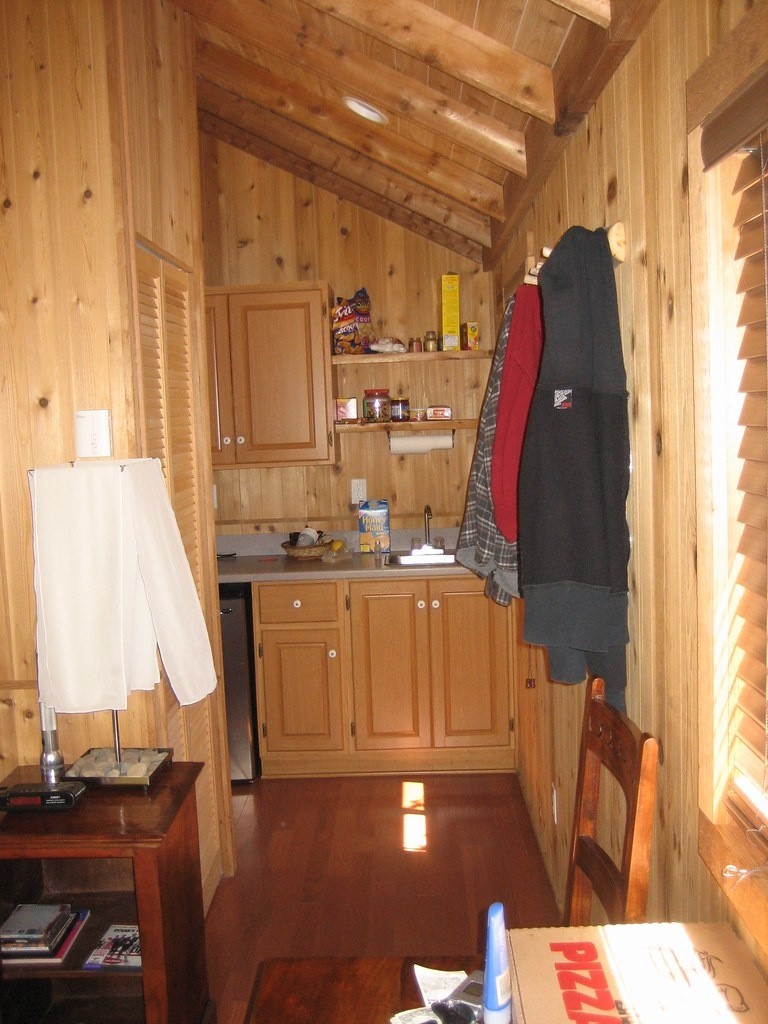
[281,532,333,561]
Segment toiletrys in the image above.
[483,902,512,1024]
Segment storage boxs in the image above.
[359,499,392,553]
[437,271,460,350]
[336,398,358,421]
[505,923,767,1024]
[460,322,479,350]
[426,406,452,421]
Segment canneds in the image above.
[363,388,393,423]
[390,396,411,422]
[408,408,427,421]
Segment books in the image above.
[0,903,90,963]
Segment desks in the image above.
[245,956,484,1024]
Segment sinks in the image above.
[384,553,456,566]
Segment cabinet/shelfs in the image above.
[345,575,515,776]
[0,762,219,1024]
[0,0,238,918]
[204,280,341,471]
[331,349,495,432]
[251,578,351,780]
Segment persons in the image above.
[97,933,138,959]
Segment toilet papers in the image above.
[390,435,452,454]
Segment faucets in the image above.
[424,505,432,549]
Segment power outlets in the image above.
[351,479,368,504]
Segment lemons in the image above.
[332,540,344,552]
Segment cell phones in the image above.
[446,970,485,1024]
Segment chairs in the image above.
[563,675,659,926]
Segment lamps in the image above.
[27,458,219,798]
